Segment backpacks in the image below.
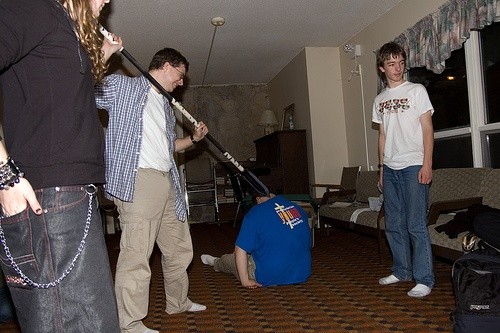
[450,247,500,333]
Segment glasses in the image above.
[173,66,185,80]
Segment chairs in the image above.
[311,166,362,236]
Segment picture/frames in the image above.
[281,104,296,130]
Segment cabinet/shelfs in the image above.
[254,130,309,195]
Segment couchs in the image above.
[319,168,500,261]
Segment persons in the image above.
[200,182,313,289]
[371,41,436,298]
[1,0,122,333]
[93,31,209,333]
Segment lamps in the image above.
[257,109,278,135]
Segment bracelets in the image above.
[0,156,24,191]
[191,134,198,144]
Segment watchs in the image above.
[378,163,384,169]
